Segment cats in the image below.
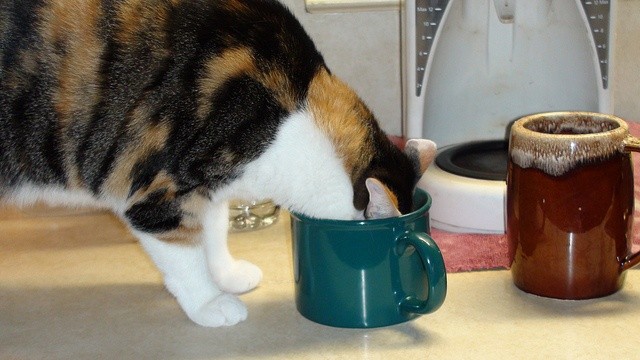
[0,0,438,328]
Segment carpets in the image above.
[383,119,640,273]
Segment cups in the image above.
[289,187,447,329]
[504,111,636,299]
